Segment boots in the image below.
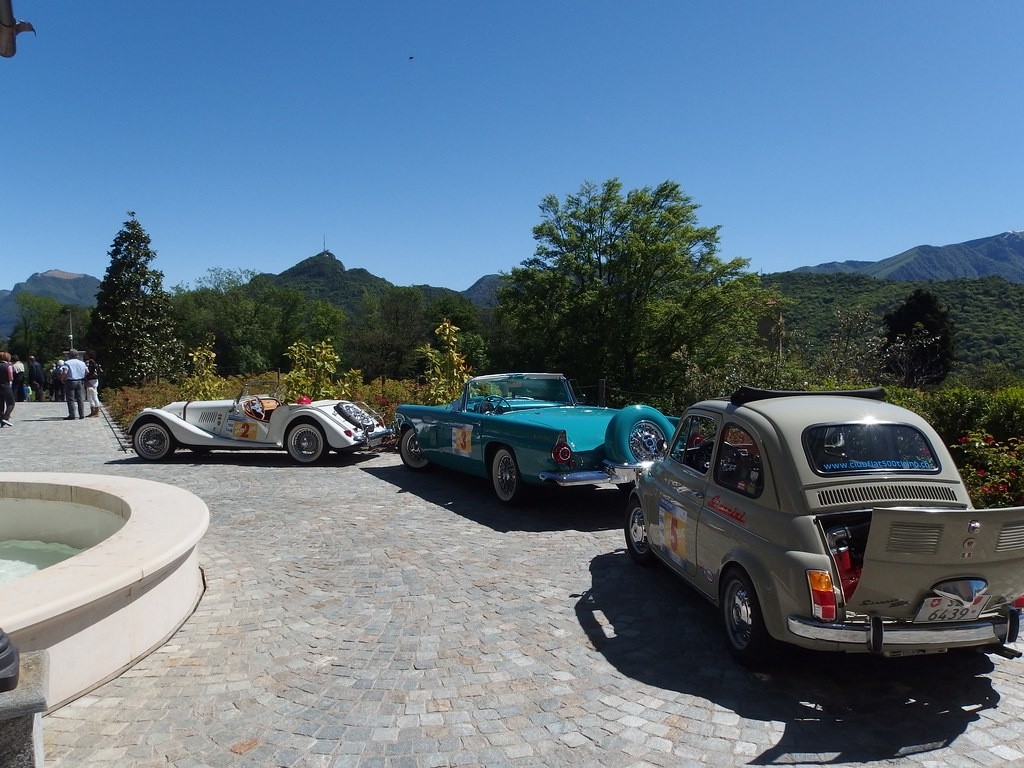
[93,406,100,415]
[85,406,96,417]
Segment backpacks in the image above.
[46,369,53,384]
[52,366,62,381]
[88,365,98,379]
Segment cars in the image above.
[623,386,1024,667]
[126,368,396,465]
[392,373,704,513]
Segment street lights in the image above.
[67,309,73,349]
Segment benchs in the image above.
[270,404,289,423]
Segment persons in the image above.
[0,348,65,401]
[0,352,15,428]
[84,349,100,418]
[61,348,89,420]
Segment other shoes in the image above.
[2,419,13,426]
